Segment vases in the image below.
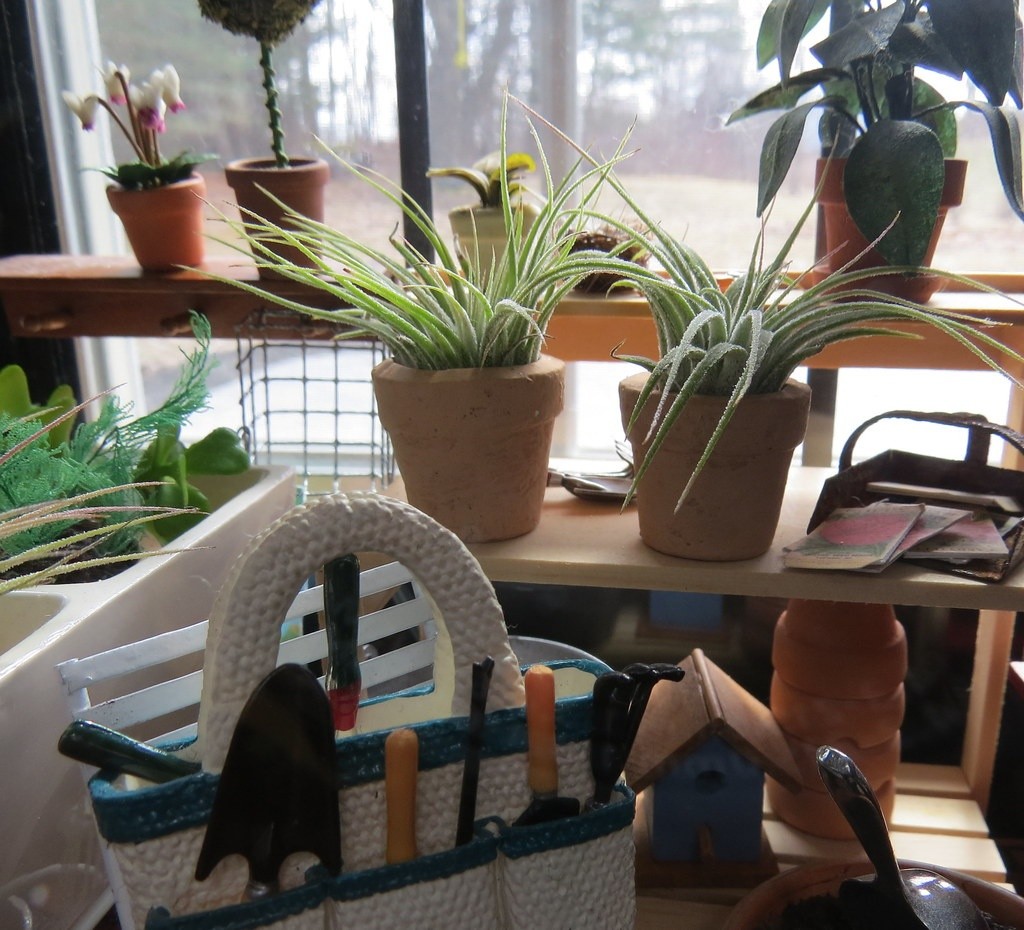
[107,173,206,272]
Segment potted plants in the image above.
[0,309,299,929]
[426,149,546,271]
[514,95,1024,557]
[725,1,1023,301]
[178,90,654,543]
[203,0,330,277]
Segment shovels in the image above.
[194,659,344,903]
[513,665,581,830]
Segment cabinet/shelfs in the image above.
[0,255,1024,930]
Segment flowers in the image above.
[64,55,220,189]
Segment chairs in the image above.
[54,545,442,930]
